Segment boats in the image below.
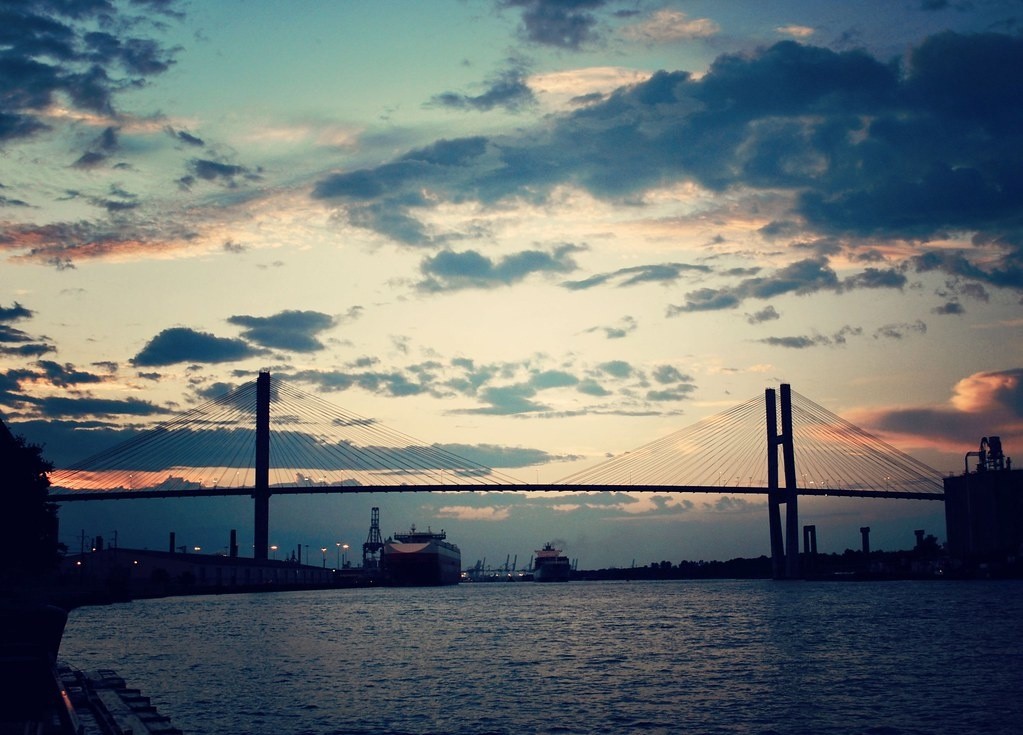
[380,534,461,586]
[533,542,571,582]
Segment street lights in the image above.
[335,542,341,569]
[271,546,278,560]
[343,545,349,567]
[321,548,326,567]
